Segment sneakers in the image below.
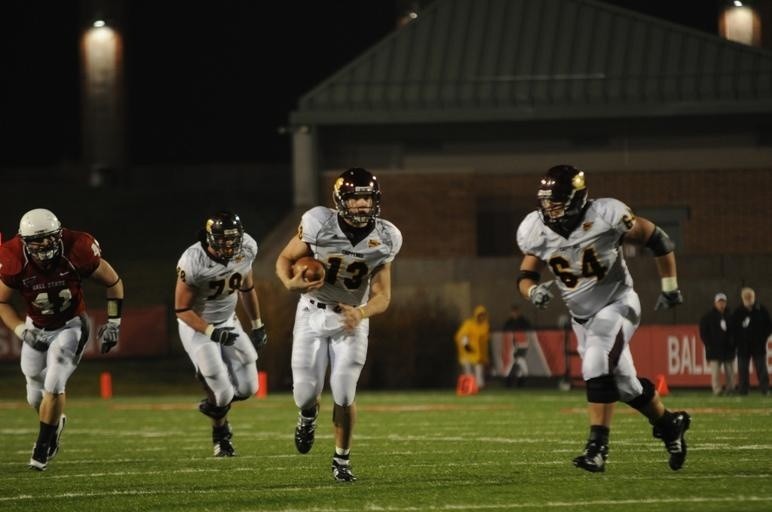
[46,413,66,460]
[714,387,772,396]
[653,410,690,470]
[295,399,320,454]
[332,457,356,482]
[213,431,235,457]
[572,437,609,473]
[30,442,51,471]
[200,399,230,419]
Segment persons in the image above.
[172,208,269,457]
[454,305,532,390]
[0,207,124,469]
[699,293,737,396]
[515,163,692,472]
[274,168,403,483]
[729,286,772,394]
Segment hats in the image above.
[715,293,726,302]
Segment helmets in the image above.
[18,209,63,264]
[535,164,590,223]
[332,168,381,225]
[206,209,244,258]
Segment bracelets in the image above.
[107,298,124,318]
[527,284,537,298]
[357,307,364,318]
[204,324,214,337]
[14,324,27,338]
[661,277,678,292]
[249,318,262,328]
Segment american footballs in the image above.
[293,256,326,281]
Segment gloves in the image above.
[654,290,682,312]
[22,328,49,352]
[530,279,555,310]
[96,322,120,353]
[252,325,267,347]
[211,326,240,347]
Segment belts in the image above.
[32,321,67,332]
[309,299,357,314]
